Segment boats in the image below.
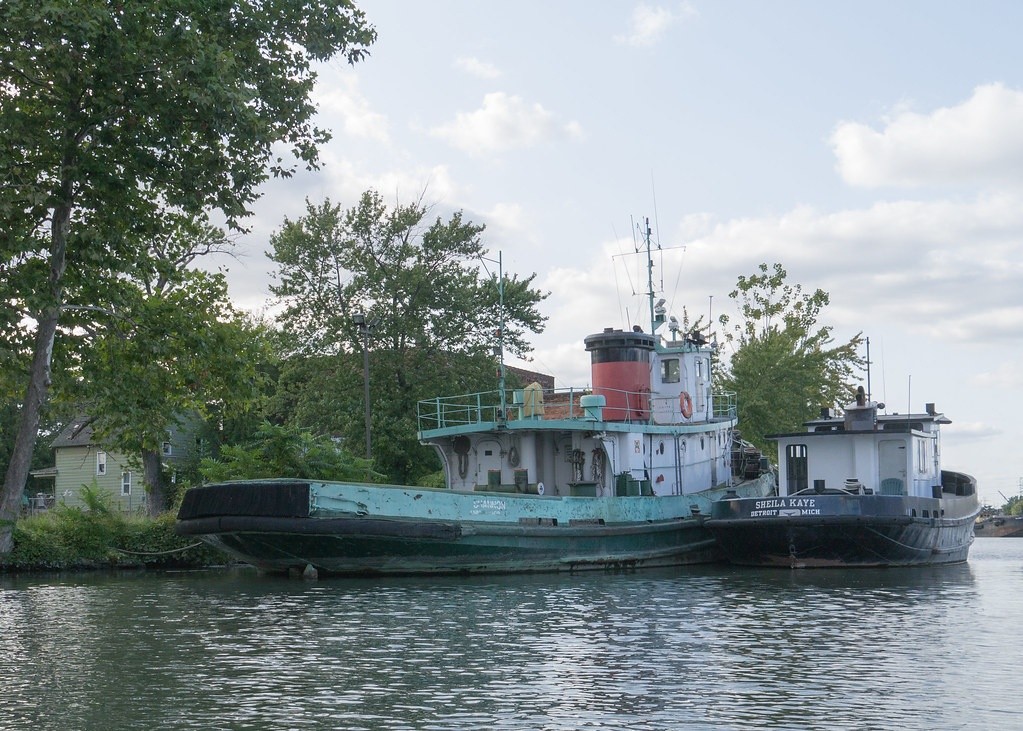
[711,335,978,567]
[175,211,776,582]
[971,512,1022,538]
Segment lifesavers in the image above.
[680,390,692,418]
